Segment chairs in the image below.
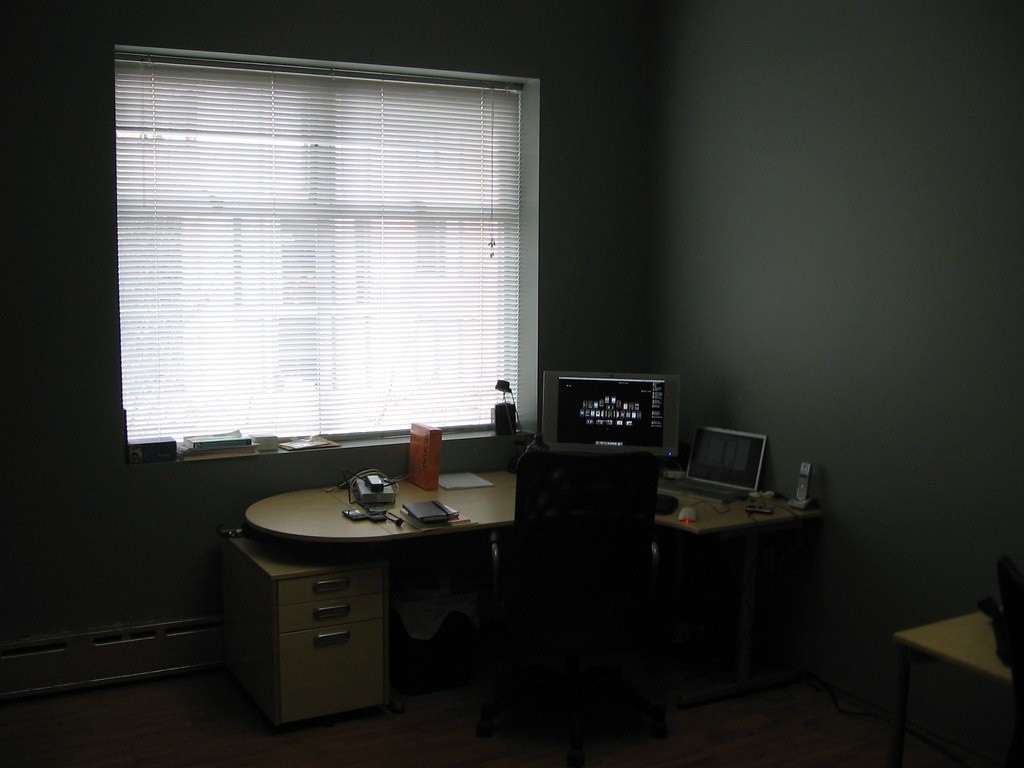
[476,450,666,768]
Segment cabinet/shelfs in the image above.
[222,537,389,728]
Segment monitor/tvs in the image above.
[542,370,680,458]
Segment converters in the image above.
[364,475,384,492]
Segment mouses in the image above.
[679,507,698,522]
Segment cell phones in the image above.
[343,509,368,521]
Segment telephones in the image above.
[788,461,817,510]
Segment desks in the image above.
[886,602,1024,768]
[242,467,819,709]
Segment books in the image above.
[403,499,471,525]
[183,429,252,449]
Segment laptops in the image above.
[657,425,767,505]
